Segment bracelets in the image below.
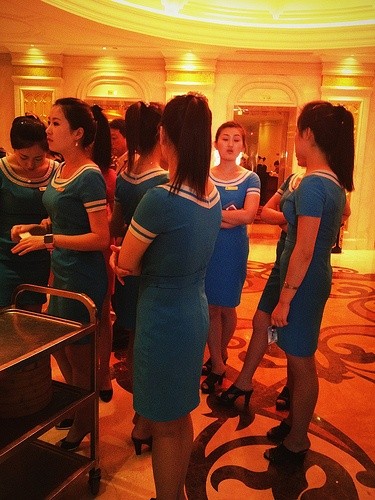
[283,282,299,290]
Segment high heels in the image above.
[200,356,227,374]
[130,434,154,456]
[56,431,93,451]
[202,371,225,391]
[276,386,293,409]
[98,389,114,403]
[264,442,307,463]
[266,421,294,442]
[55,418,76,429]
[217,383,254,408]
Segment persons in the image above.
[107,117,141,176]
[256,156,268,206]
[0,111,61,314]
[10,96,113,449]
[273,161,279,176]
[46,149,65,163]
[210,148,309,412]
[107,99,172,455]
[331,188,352,254]
[108,90,223,500]
[264,99,356,471]
[83,140,117,402]
[198,114,262,394]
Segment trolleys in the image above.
[0,284,104,500]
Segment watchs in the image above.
[43,234,56,252]
